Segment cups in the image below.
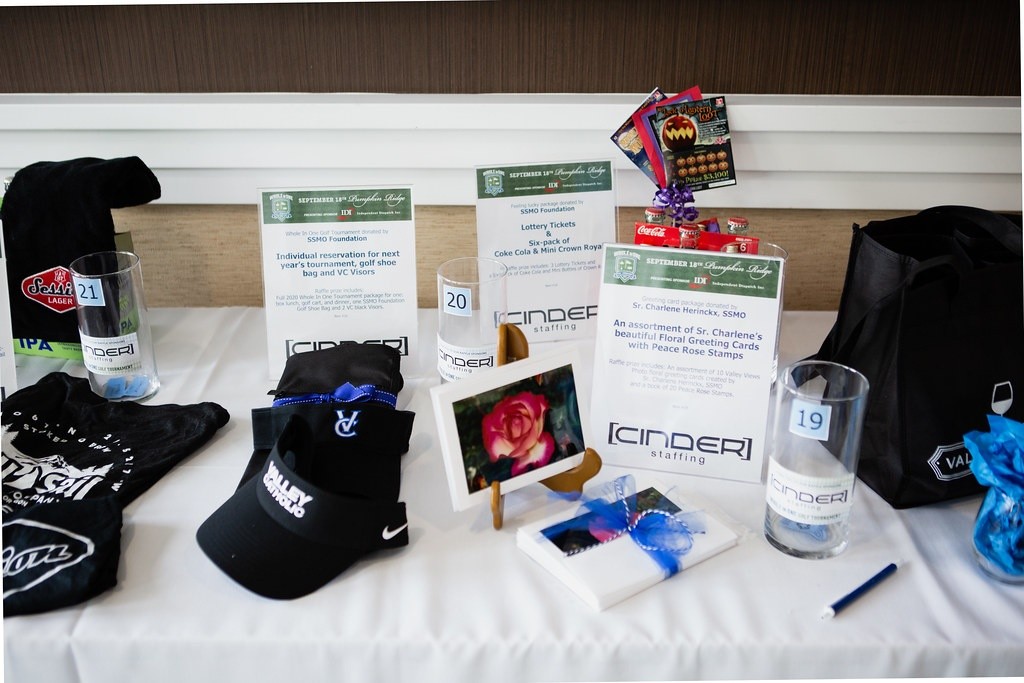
[764,361,869,561]
[719,241,788,394]
[436,257,508,384]
[70,250,161,404]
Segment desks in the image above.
[4,305,1023,676]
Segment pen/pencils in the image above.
[819,558,903,619]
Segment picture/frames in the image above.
[430,337,597,514]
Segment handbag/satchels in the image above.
[771,206,1024,509]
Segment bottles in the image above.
[643,206,750,255]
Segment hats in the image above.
[195,406,417,600]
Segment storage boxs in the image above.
[12,230,140,362]
